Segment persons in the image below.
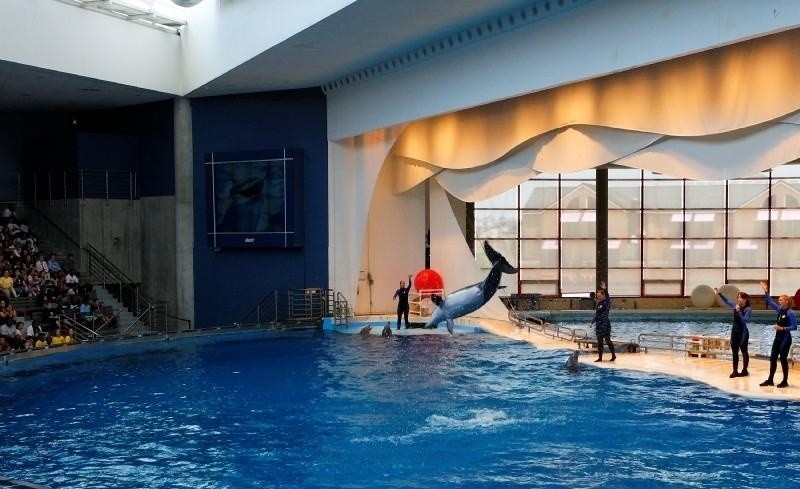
[761,278,798,388]
[589,280,618,362]
[0,205,112,354]
[392,273,414,330]
[713,287,752,378]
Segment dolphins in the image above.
[565,350,581,367]
[359,325,373,334]
[423,238,521,334]
[382,321,393,337]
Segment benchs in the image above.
[8,296,114,321]
